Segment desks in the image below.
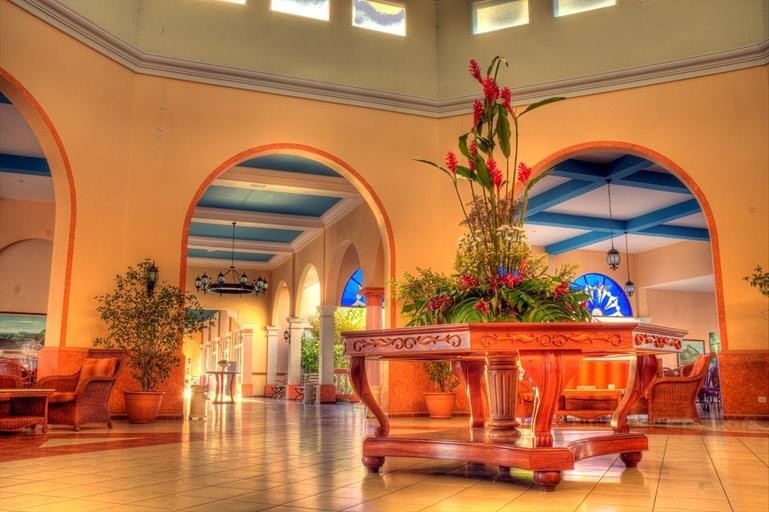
[207,370,241,404]
[340,320,689,492]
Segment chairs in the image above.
[0,349,127,431]
[647,352,722,425]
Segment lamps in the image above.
[194,222,269,299]
[145,260,159,297]
[604,178,635,298]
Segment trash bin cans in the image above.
[189,385,210,420]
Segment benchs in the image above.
[270,373,338,405]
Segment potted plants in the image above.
[421,359,460,419]
[91,257,218,424]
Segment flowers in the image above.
[381,53,598,327]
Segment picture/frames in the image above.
[0,311,48,353]
[677,338,705,368]
